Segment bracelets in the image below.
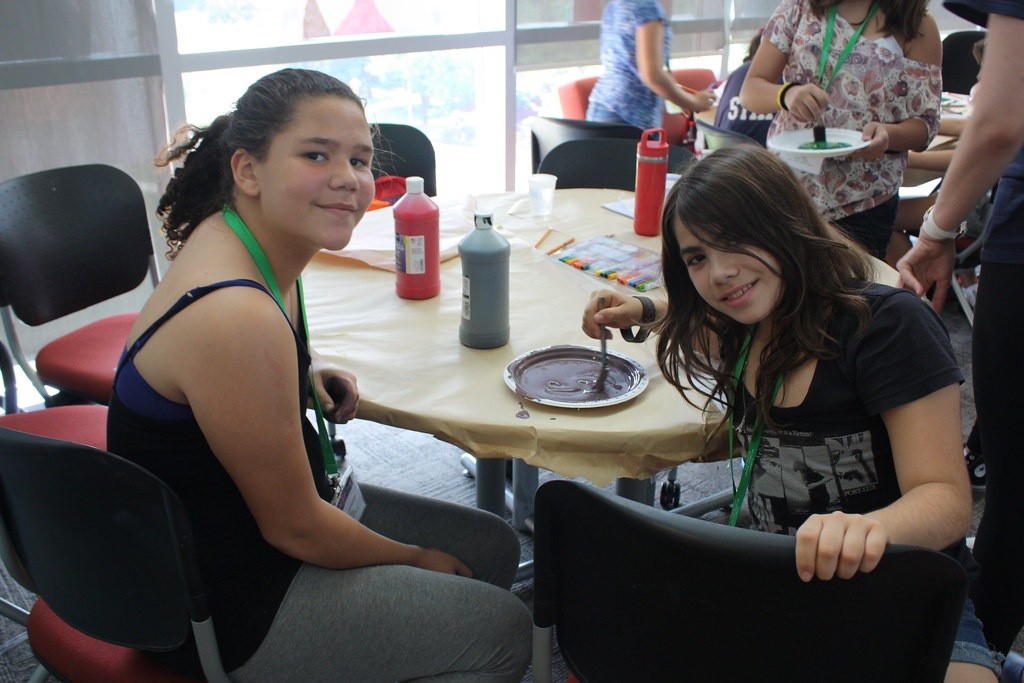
[923,204,967,241]
[620,296,656,343]
[777,81,802,112]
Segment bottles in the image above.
[457,211,511,350]
[391,175,442,301]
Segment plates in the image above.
[766,127,869,158]
[503,344,649,409]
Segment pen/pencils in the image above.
[706,83,716,94]
[535,229,552,249]
[559,254,666,289]
[548,237,575,256]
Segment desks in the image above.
[302,186,905,599]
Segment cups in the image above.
[527,172,558,217]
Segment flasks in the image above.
[633,127,669,238]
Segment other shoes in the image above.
[962,441,987,490]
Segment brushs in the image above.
[812,78,827,147]
[592,295,610,391]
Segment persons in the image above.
[897,0,1024,655]
[582,142,1004,683]
[885,117,999,270]
[107,63,534,683]
[585,0,717,142]
[739,1,943,264]
[714,32,785,149]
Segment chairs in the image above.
[371,123,437,198]
[941,30,986,95]
[0,164,349,683]
[532,478,968,683]
[528,68,765,188]
